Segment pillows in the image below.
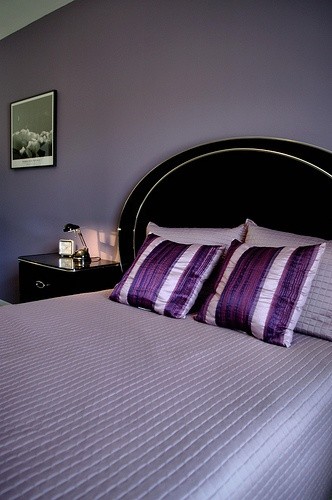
[194,238,326,348]
[245,220,332,343]
[146,222,248,259]
[109,232,225,319]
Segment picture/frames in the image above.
[9,89,57,169]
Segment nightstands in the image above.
[18,253,121,303]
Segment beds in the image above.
[0,136,332,500]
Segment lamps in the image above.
[63,223,90,261]
